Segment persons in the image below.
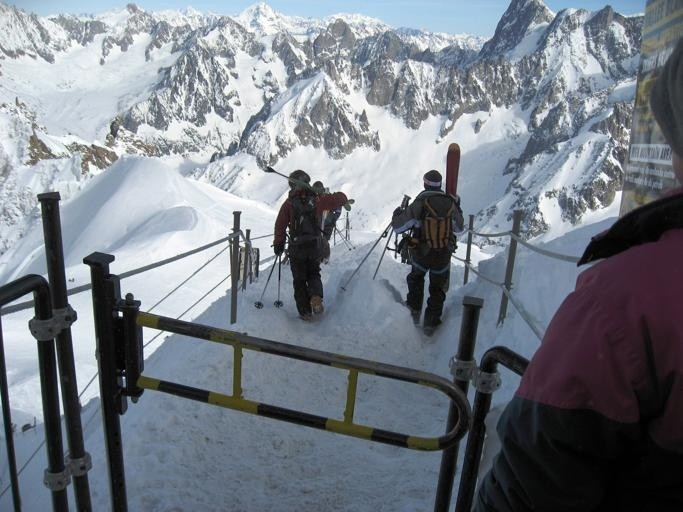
[313,181,341,264]
[392,171,464,335]
[273,170,350,322]
[470,34,682,512]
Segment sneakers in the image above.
[302,315,312,321]
[424,319,441,329]
[310,296,323,313]
[407,303,421,314]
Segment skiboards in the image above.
[446,143,460,291]
[263,166,354,211]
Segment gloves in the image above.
[449,193,460,208]
[393,207,404,217]
[274,245,284,256]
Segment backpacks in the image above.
[324,206,341,226]
[420,196,454,249]
[288,194,329,262]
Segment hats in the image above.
[313,181,325,193]
[290,171,310,188]
[651,36,683,158]
[424,170,441,190]
